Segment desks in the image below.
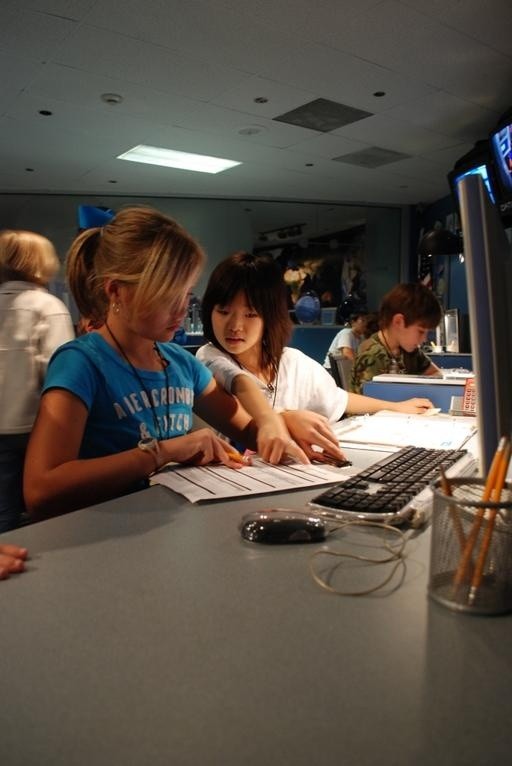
[0,414,511,766]
[363,354,474,414]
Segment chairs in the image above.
[330,353,352,391]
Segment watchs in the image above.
[136,435,162,474]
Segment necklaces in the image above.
[236,358,274,391]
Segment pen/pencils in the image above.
[226,451,250,463]
[439,435,512,607]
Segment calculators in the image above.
[463,378,478,416]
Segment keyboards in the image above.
[307,445,475,520]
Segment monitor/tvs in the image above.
[450,174,505,486]
[447,147,497,215]
[486,114,512,193]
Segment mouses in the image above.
[239,508,323,546]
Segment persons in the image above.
[1,539,29,579]
[22,207,314,512]
[194,250,439,468]
[347,281,443,417]
[1,228,78,535]
[322,308,369,389]
